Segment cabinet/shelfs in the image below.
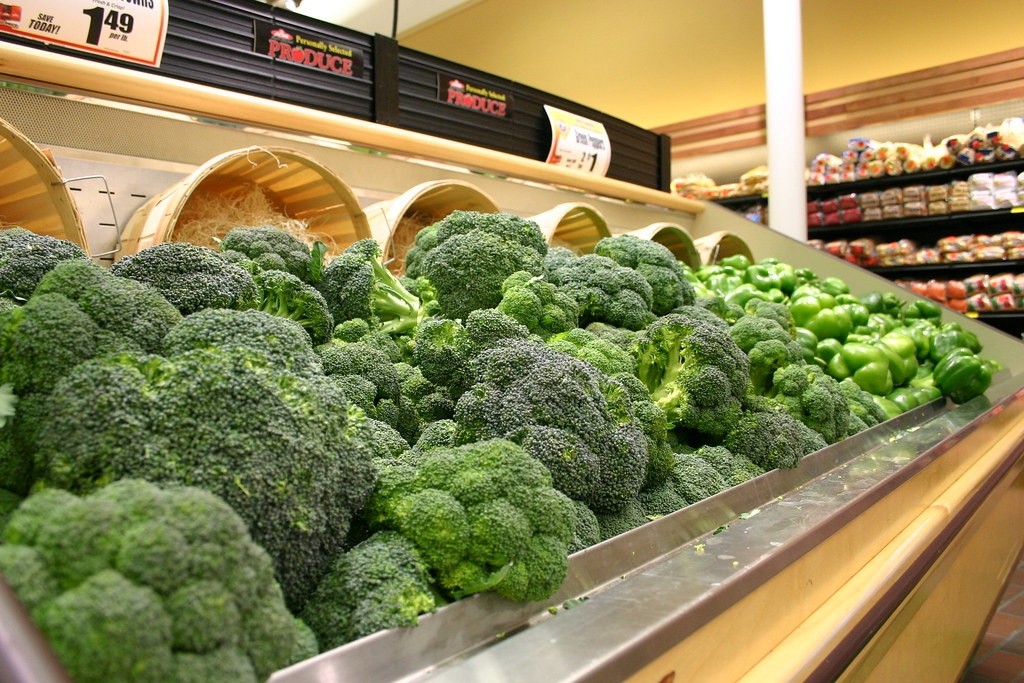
[706,159,1024,344]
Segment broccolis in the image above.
[0,210,888,683]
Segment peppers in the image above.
[677,253,1003,421]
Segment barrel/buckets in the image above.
[524,202,611,249]
[363,179,503,271]
[0,118,122,265]
[614,222,755,268]
[113,145,373,264]
